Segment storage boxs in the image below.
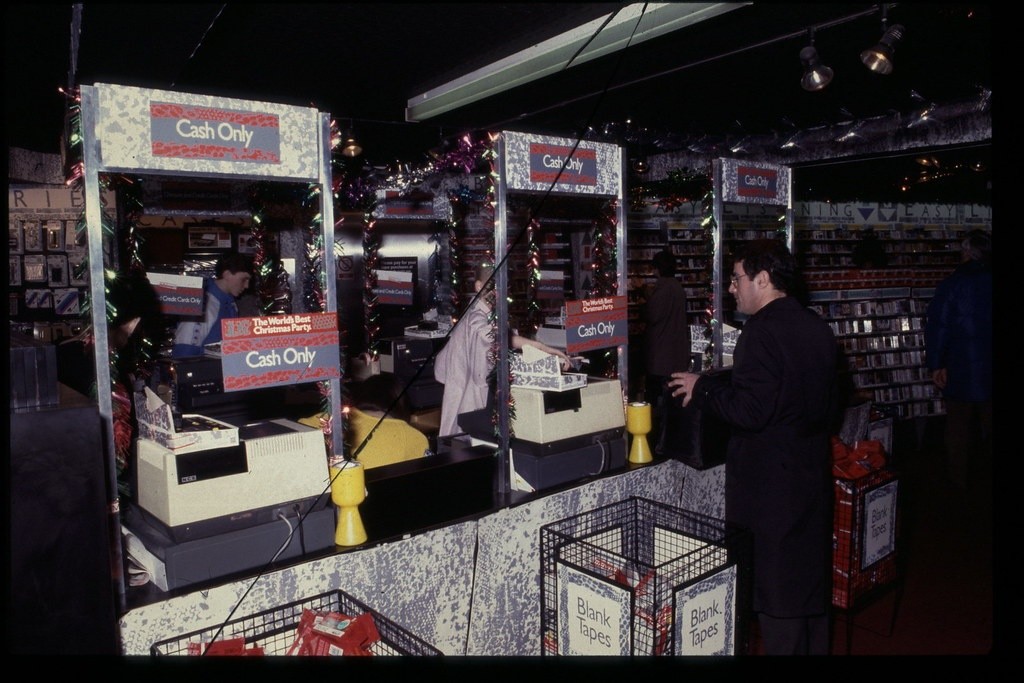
[134,392,239,455]
[509,351,588,392]
[404,321,451,338]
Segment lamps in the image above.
[342,119,363,157]
[859,3,907,75]
[404,0,755,122]
[799,24,834,92]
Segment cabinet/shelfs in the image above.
[455,221,993,423]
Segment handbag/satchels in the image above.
[654,367,734,470]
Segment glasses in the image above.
[730,272,755,284]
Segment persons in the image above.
[638,251,689,455]
[668,238,839,655]
[435,267,574,437]
[926,229,992,545]
[171,253,255,358]
